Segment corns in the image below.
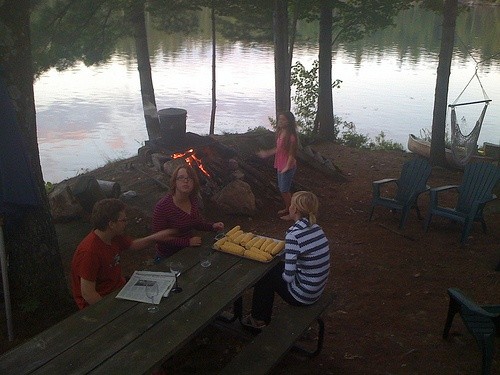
[214,225,285,260]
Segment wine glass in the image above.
[145,281,158,310]
[169,261,182,292]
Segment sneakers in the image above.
[241,313,265,328]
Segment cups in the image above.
[200,244,212,267]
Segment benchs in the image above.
[220,286,335,375]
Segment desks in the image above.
[0,228,325,375]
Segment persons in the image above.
[241,191,330,329]
[70,198,179,312]
[153,164,225,262]
[256,112,297,220]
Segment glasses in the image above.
[176,177,192,181]
[111,217,127,223]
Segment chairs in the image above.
[366,157,434,230]
[441,288,500,375]
[424,158,500,249]
